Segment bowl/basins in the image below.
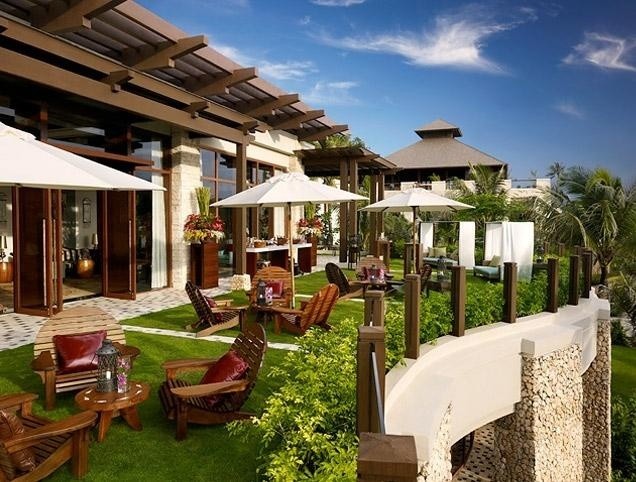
[277,238,288,245]
[293,240,304,244]
[254,242,266,248]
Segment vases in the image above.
[200,236,215,244]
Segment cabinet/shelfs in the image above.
[348,234,361,270]
[307,238,317,266]
[190,243,220,288]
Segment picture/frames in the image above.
[83,203,91,223]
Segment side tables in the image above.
[375,240,392,273]
[404,243,419,276]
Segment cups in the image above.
[378,268,384,283]
[264,287,274,306]
[114,357,129,393]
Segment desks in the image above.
[246,243,312,283]
[75,381,150,443]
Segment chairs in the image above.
[157,323,267,442]
[0,392,98,482]
[30,306,141,411]
[185,257,431,338]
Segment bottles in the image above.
[369,265,377,283]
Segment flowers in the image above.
[116,358,131,394]
[297,205,323,239]
[183,186,226,244]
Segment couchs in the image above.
[423,252,459,267]
[474,261,501,283]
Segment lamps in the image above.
[89,339,122,393]
[0,235,7,262]
[92,233,98,249]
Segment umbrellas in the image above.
[353,188,477,273]
[0,117,166,194]
[207,171,368,309]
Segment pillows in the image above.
[53,330,107,374]
[428,247,446,258]
[0,409,36,474]
[200,350,249,408]
[489,256,500,266]
